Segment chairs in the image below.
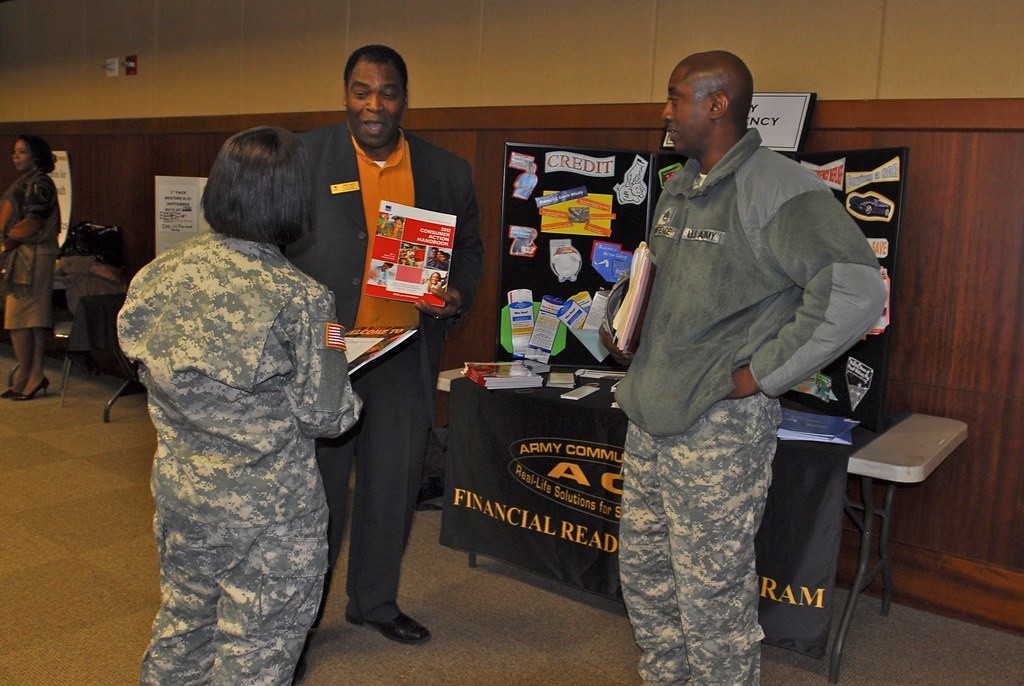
[55,320,73,407]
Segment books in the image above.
[611,240,658,361]
[461,357,580,390]
[362,198,457,309]
[771,405,861,445]
[341,323,419,382]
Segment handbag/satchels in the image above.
[69,221,122,266]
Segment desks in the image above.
[8,276,128,388]
[66,293,141,423]
[438,368,969,686]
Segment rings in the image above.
[433,315,440,320]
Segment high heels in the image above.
[1,390,21,399]
[8,377,50,400]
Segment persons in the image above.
[280,41,481,643]
[1,132,63,400]
[114,123,366,686]
[368,213,450,301]
[615,49,886,686]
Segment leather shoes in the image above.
[346,608,432,644]
